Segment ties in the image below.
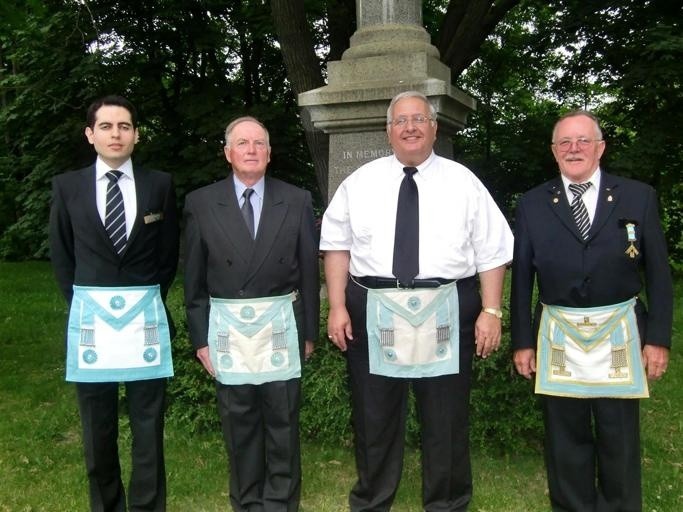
[105,172,127,255]
[569,183,592,241]
[241,189,254,240]
[392,167,419,287]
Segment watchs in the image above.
[481,308,503,318]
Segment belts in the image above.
[377,278,441,290]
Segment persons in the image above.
[511,111,673,512]
[318,91,515,512]
[180,116,319,512]
[49,94,180,512]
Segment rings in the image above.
[662,371,666,374]
[328,336,332,340]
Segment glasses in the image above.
[552,137,604,152]
[389,115,433,125]
